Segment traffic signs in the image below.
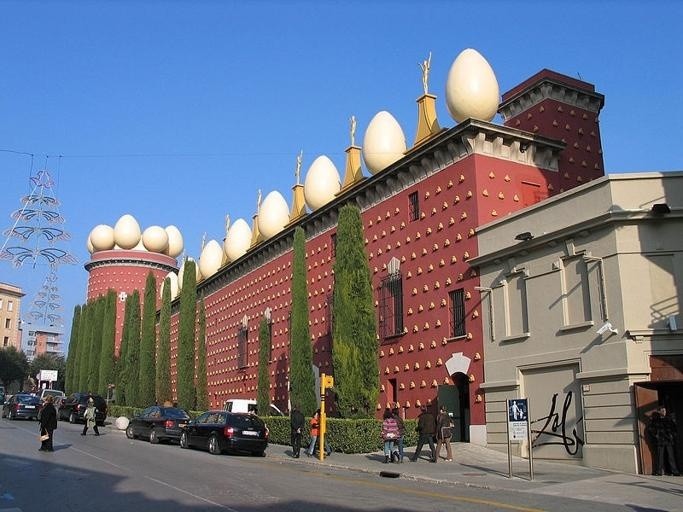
[507,398,527,441]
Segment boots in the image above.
[382,455,389,463]
[394,451,401,463]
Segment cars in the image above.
[1,386,66,420]
[178,410,269,456]
[125,405,190,445]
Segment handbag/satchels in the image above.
[39,432,49,441]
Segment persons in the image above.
[291,406,305,459]
[410,405,431,461]
[81,397,100,436]
[381,408,401,463]
[306,412,320,457]
[648,405,681,475]
[389,409,405,464]
[435,404,452,462]
[38,394,57,452]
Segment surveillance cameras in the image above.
[596,322,612,336]
[670,315,677,331]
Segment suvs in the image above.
[57,393,107,426]
